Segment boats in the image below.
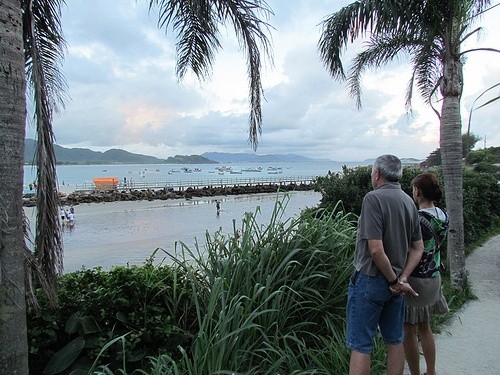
[92,177,118,182]
[138,166,282,175]
[102,169,107,172]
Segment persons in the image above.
[61,205,75,227]
[214,200,221,217]
[403,174,449,374]
[344,154,424,375]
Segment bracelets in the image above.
[388,276,398,286]
